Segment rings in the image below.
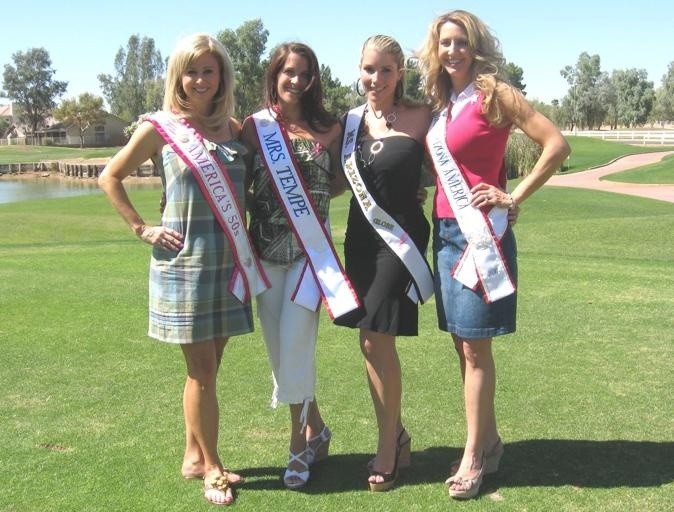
[161,239,166,245]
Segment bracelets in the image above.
[507,190,517,210]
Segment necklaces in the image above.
[355,100,399,168]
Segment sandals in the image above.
[182,463,245,486]
[202,467,233,505]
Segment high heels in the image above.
[284,446,309,489]
[445,451,486,499]
[367,446,399,492]
[449,437,505,476]
[366,429,411,473]
[306,426,332,461]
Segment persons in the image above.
[99,35,249,506]
[160,43,427,491]
[417,9,571,498]
[334,32,434,492]
